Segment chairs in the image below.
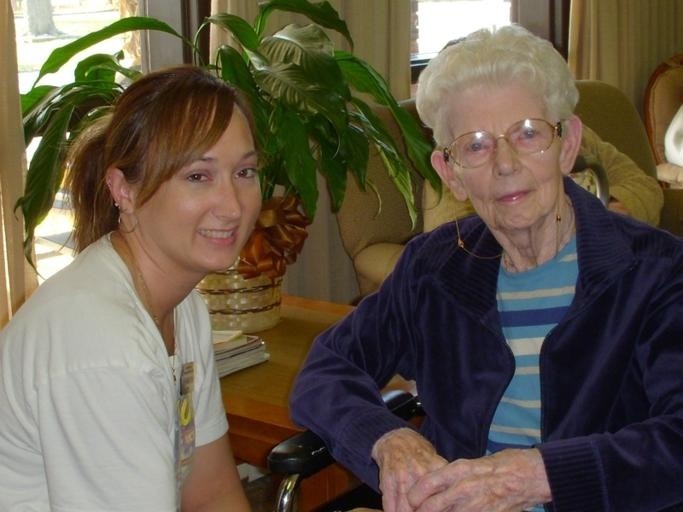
[324,78,682,303]
[643,51,683,188]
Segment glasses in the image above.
[442,115,563,169]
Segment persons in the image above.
[290,25,683,511]
[422,117,663,234]
[0,69,263,512]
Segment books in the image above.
[203,321,269,377]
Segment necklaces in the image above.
[114,227,190,382]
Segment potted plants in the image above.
[12,1,445,336]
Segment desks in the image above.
[215,295,422,511]
